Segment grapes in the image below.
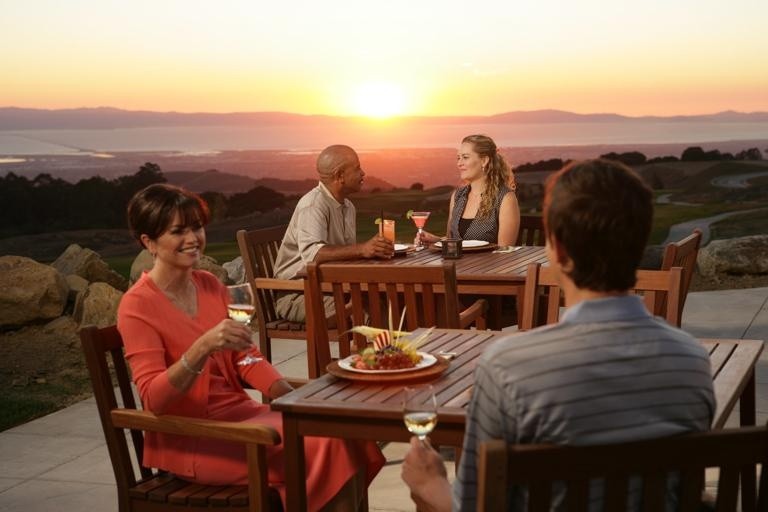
[368,351,416,370]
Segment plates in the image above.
[336,350,437,373]
[433,240,490,249]
[393,243,408,251]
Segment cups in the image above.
[439,239,463,260]
[377,218,395,252]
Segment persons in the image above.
[115,183,386,511]
[414,133,521,250]
[399,158,716,512]
[273,145,396,330]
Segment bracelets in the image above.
[178,351,204,378]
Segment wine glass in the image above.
[401,383,438,444]
[225,281,262,367]
[409,211,431,251]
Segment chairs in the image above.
[304,262,490,377]
[521,261,685,336]
[78,325,311,512]
[460,211,546,327]
[474,423,767,512]
[236,225,355,379]
[644,228,708,325]
